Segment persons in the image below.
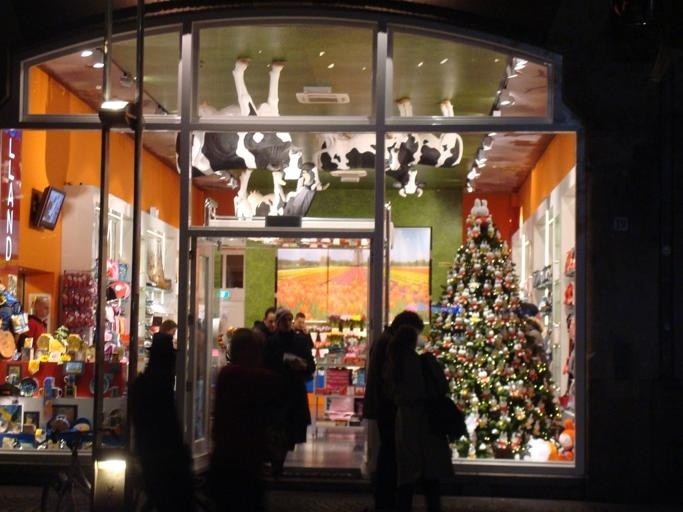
[515,302,541,320]
[522,314,547,349]
[361,310,466,512]
[16,293,50,354]
[211,306,316,512]
[128,320,198,512]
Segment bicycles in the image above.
[43,423,213,512]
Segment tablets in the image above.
[63,360,86,375]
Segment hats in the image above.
[276,307,292,326]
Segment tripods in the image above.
[54,438,92,512]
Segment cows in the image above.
[416,97,465,169]
[171,54,330,194]
[231,169,296,223]
[310,96,424,199]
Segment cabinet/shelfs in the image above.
[61,184,179,394]
[314,352,366,439]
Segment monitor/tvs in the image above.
[32,184,66,232]
